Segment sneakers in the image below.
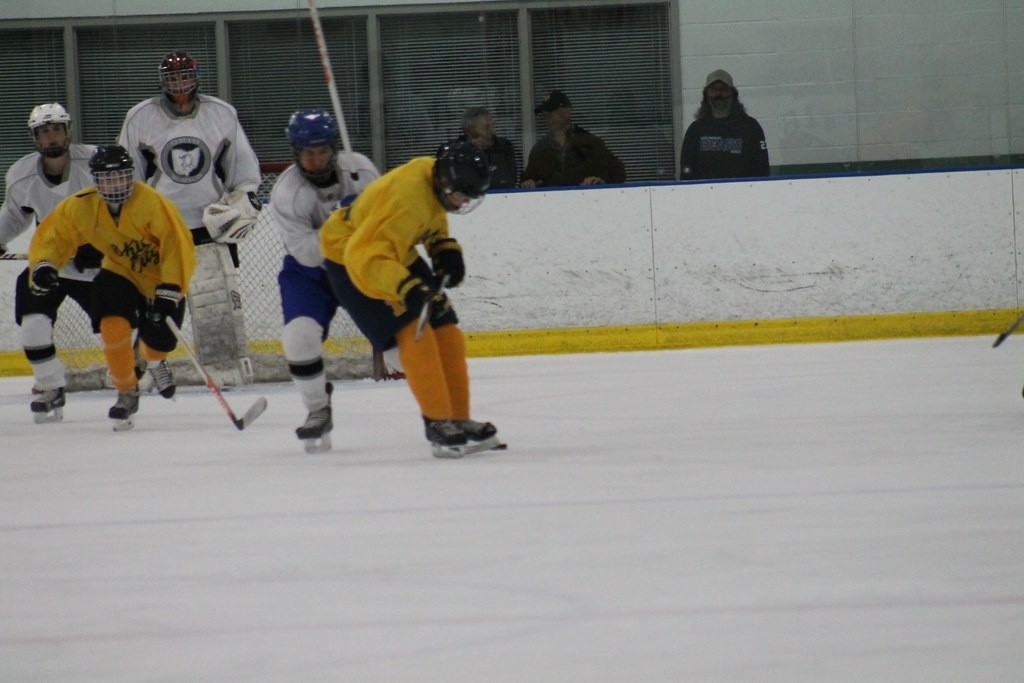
[453,419,507,450]
[147,359,176,403]
[296,382,334,454]
[108,384,140,432]
[31,385,66,423]
[422,416,467,458]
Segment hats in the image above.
[534,91,571,114]
[704,70,733,88]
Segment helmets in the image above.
[285,108,339,178]
[431,142,492,216]
[89,144,134,201]
[158,51,199,102]
[27,103,71,159]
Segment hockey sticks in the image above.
[0,252,28,260]
[310,0,361,194]
[416,271,452,346]
[164,316,268,432]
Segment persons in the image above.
[117,50,262,396]
[269,108,406,453]
[0,104,152,398]
[457,107,516,189]
[319,137,508,457]
[680,69,770,180]
[28,144,196,432]
[519,91,626,189]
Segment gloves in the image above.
[428,238,465,287]
[72,244,104,273]
[151,284,182,325]
[31,262,59,295]
[396,277,449,319]
